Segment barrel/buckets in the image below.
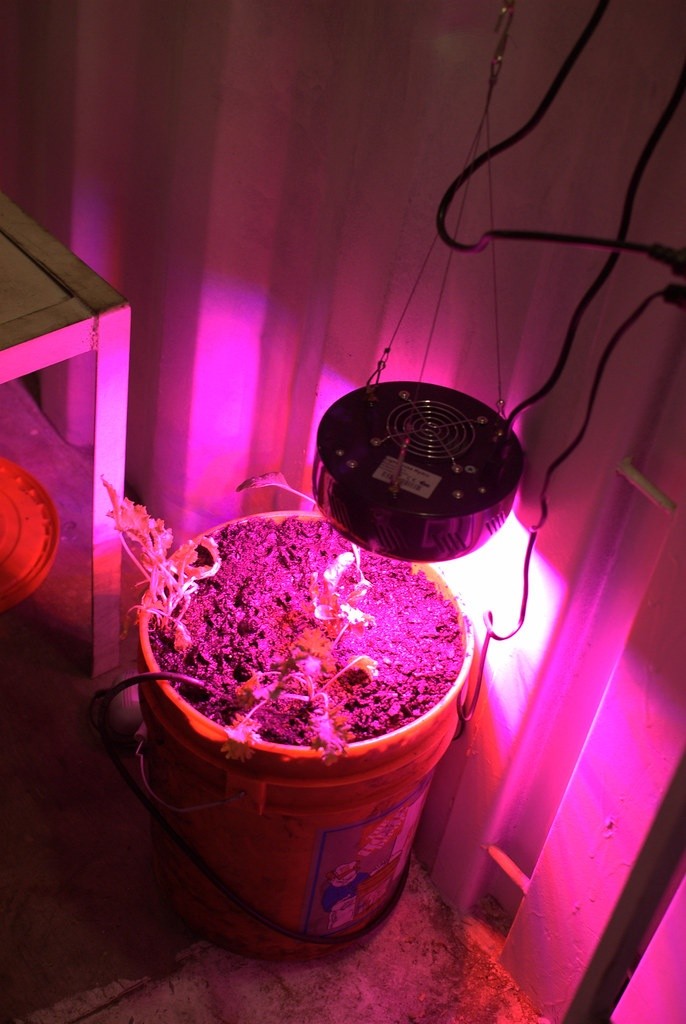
[135,509,476,967]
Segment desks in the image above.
[0,188,132,679]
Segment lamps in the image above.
[310,382,525,562]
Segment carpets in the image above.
[4,845,553,1024]
